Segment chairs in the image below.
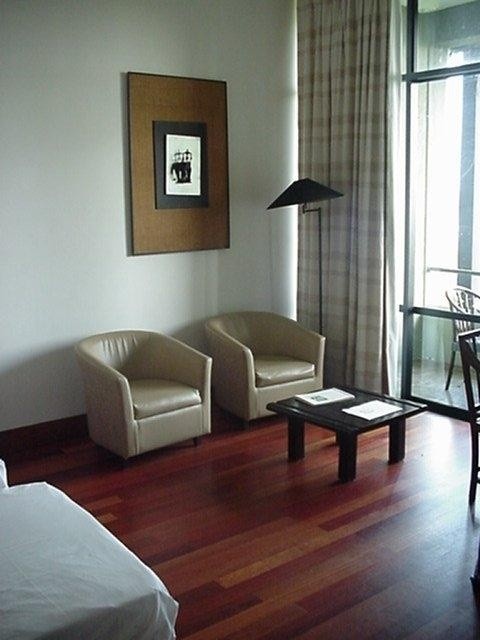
[205,311,326,431]
[457,329,480,504]
[75,329,213,471]
[445,286,480,390]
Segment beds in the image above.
[0,481,179,640]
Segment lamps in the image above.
[267,178,344,335]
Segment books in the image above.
[294,386,356,407]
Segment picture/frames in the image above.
[127,71,230,257]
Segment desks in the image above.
[266,385,428,482]
